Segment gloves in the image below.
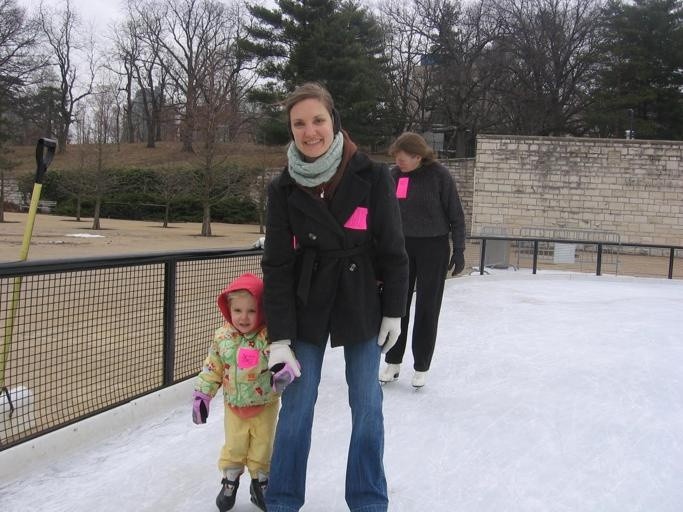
[190,389,212,426]
[447,248,465,276]
[376,316,403,355]
[262,339,303,394]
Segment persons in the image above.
[381,132,465,387]
[263,83,411,512]
[193,272,301,512]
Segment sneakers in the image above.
[377,362,400,383]
[248,476,270,512]
[214,471,241,512]
[410,370,427,388]
